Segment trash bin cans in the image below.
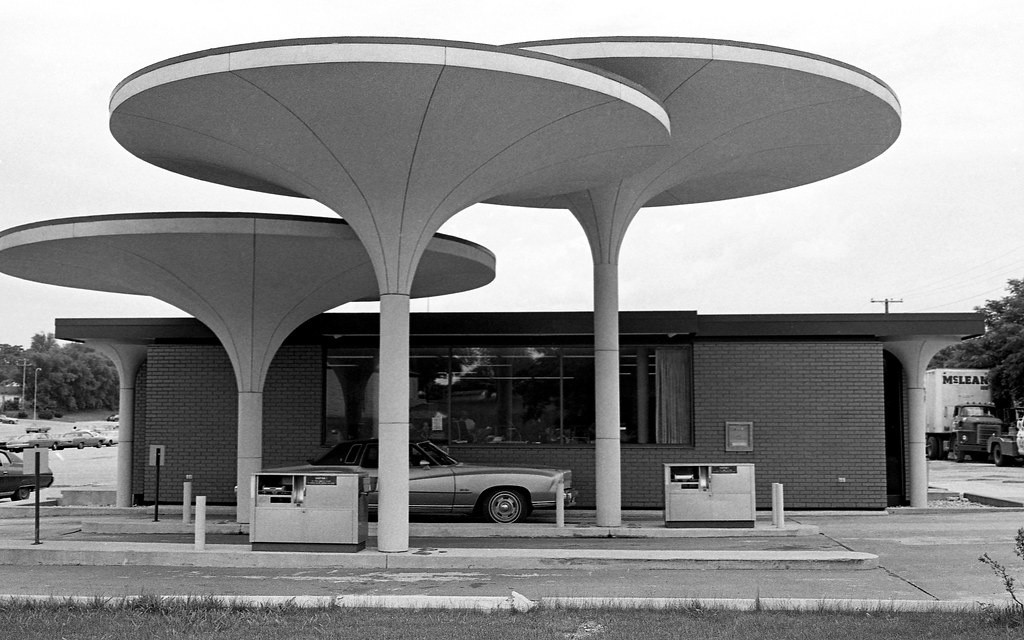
[249,473,370,553]
[662,463,756,528]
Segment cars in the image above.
[0,424,119,453]
[107,414,119,421]
[0,414,18,424]
[0,450,54,501]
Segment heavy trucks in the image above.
[925,368,1004,463]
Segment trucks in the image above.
[987,399,1024,466]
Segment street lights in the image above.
[34,367,42,420]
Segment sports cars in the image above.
[234,440,579,523]
